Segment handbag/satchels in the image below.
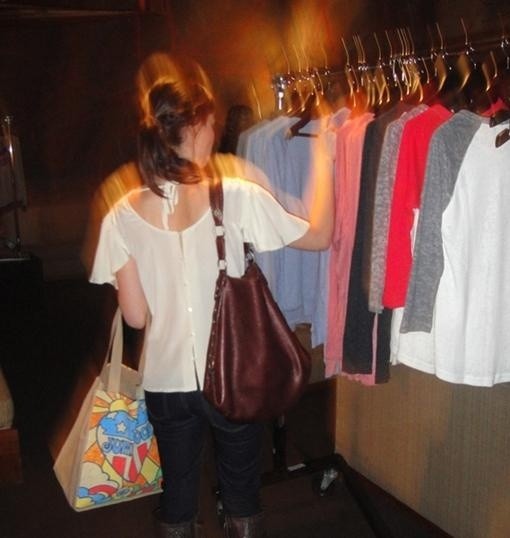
[53,361,164,512]
[204,266,311,430]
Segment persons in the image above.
[87,75,337,537]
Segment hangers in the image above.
[279,16,510,148]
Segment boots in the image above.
[151,504,204,538]
[217,506,268,538]
